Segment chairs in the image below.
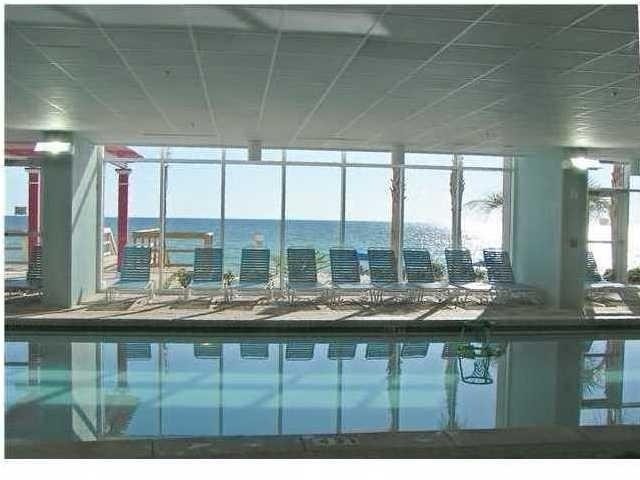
[479,246,541,307]
[4,246,46,304]
[101,245,158,306]
[580,252,638,317]
[281,247,334,312]
[367,248,417,307]
[181,247,230,308]
[229,248,274,311]
[401,248,461,310]
[327,248,378,310]
[443,246,499,311]
